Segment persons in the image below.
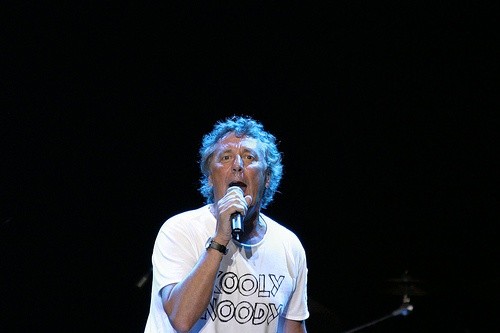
[143,115,311,333]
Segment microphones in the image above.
[226,186,244,240]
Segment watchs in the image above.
[204,236,229,255]
